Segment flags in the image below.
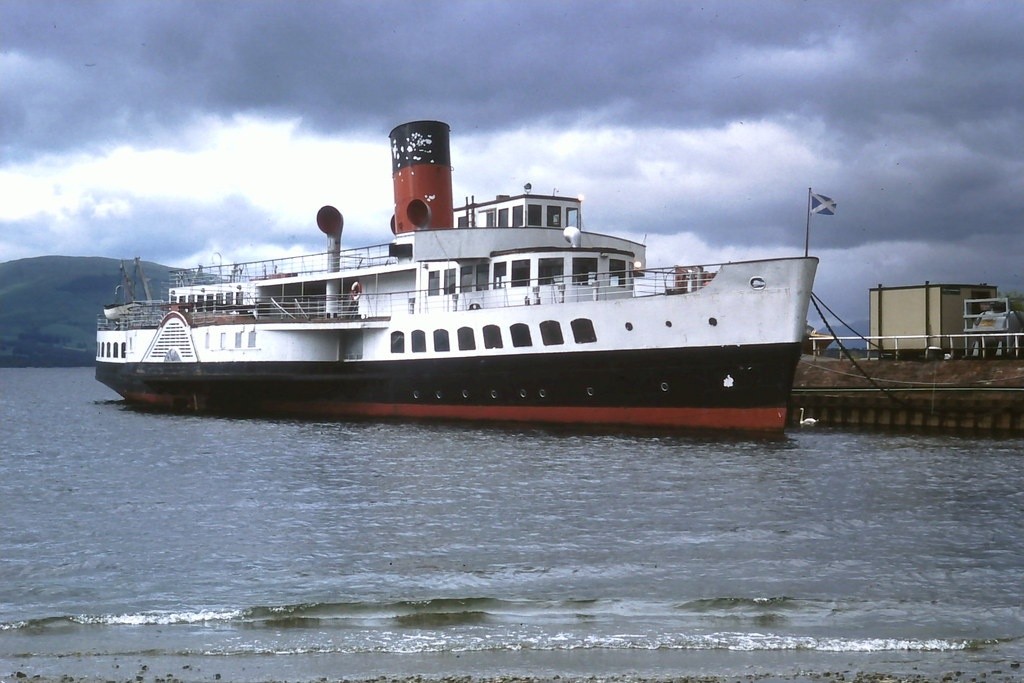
[809,191,836,215]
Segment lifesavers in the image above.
[350,281,361,301]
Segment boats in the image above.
[802,324,834,352]
[100,300,141,319]
[96,119,819,440]
